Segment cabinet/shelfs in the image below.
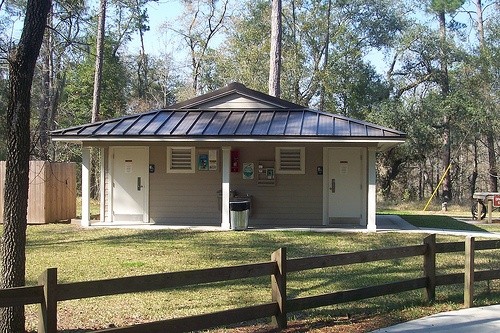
[256,161,276,187]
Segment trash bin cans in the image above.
[229,201,250,231]
